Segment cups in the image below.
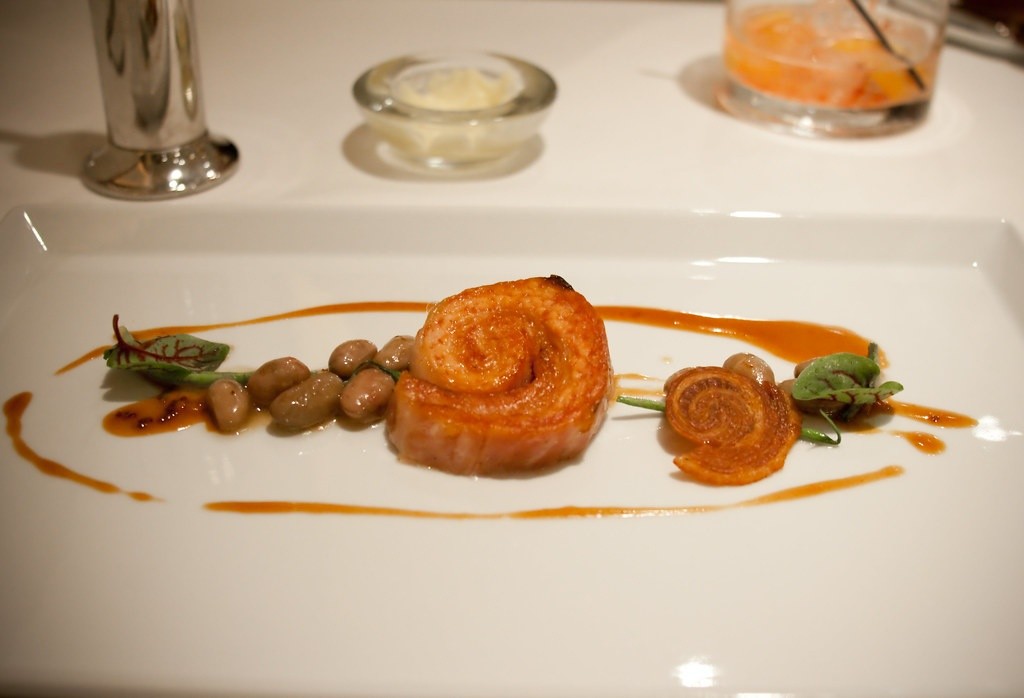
[720,0,948,142]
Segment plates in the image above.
[0,1,1024,698]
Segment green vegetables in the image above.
[613,342,904,445]
[98,315,405,387]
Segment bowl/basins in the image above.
[353,51,558,167]
